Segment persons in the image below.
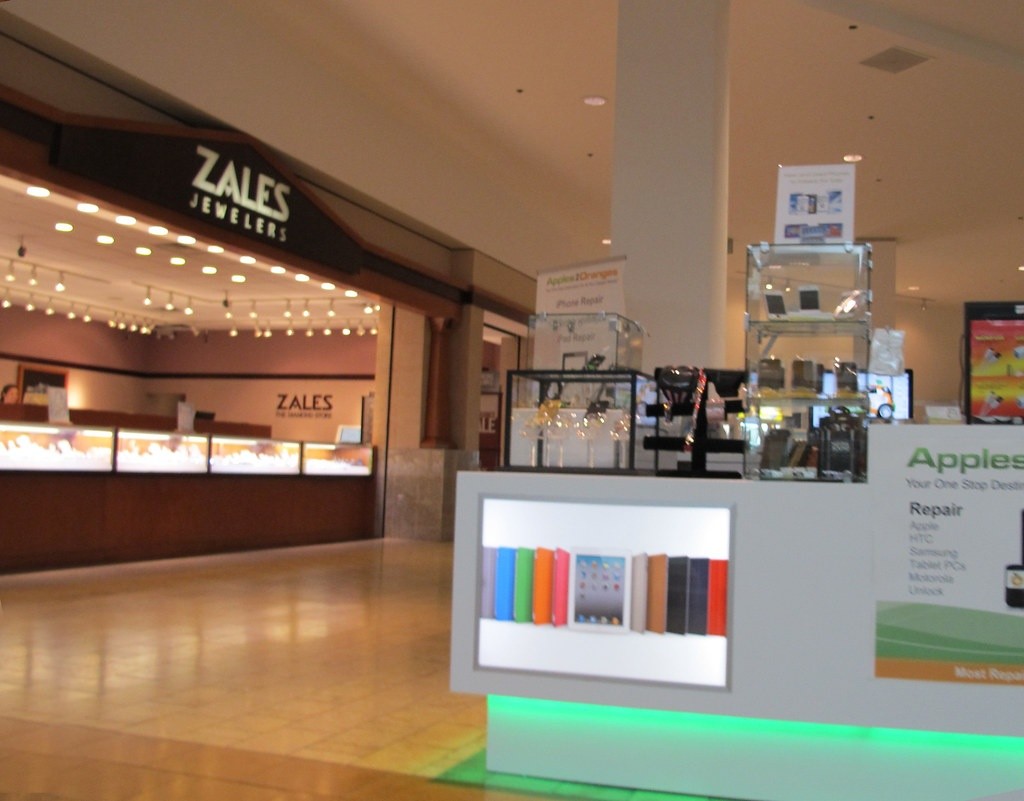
[0,383,18,403]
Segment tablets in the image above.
[482,547,729,637]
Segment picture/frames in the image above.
[16,365,71,406]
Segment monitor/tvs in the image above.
[807,368,915,437]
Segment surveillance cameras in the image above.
[222,300,228,307]
[17,246,26,257]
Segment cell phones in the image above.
[798,285,820,314]
[764,291,787,320]
[758,358,858,394]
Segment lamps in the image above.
[0,255,380,336]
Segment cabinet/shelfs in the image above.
[743,239,871,481]
